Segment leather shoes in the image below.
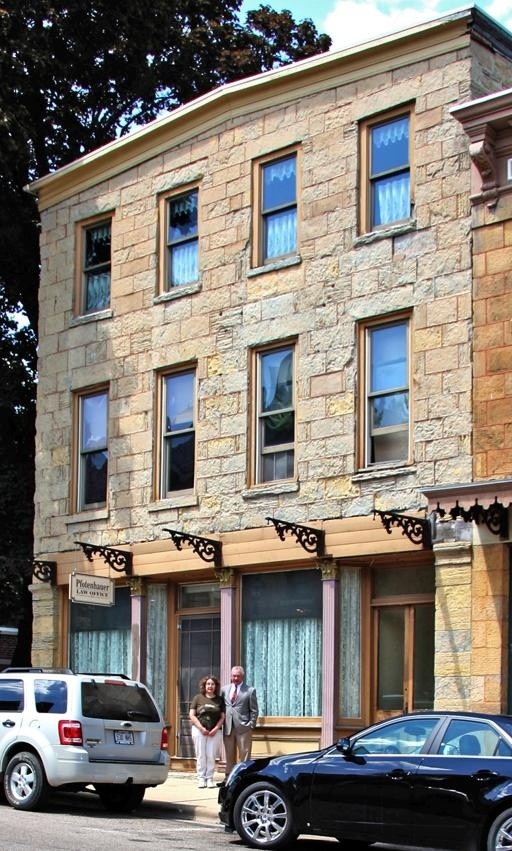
[217,781,226,787]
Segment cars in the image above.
[217,709,512,851]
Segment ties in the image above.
[231,685,237,704]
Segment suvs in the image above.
[1,665,178,814]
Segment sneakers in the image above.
[198,778,217,788]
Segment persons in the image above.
[188,676,225,788]
[216,666,259,787]
